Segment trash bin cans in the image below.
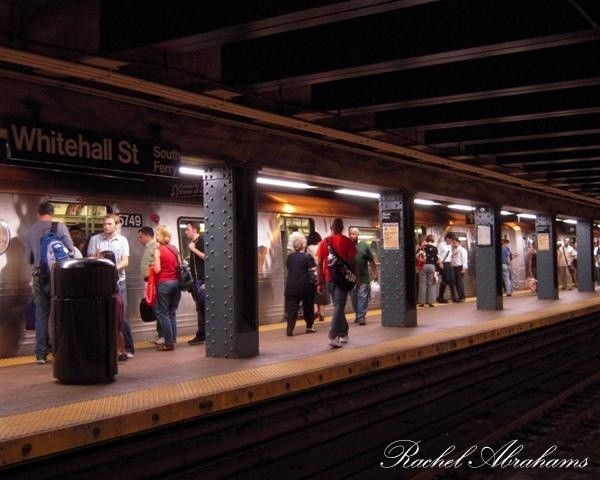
[53,257,119,385]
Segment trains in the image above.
[0,169,599,363]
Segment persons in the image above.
[21,203,599,366]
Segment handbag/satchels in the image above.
[191,278,205,302]
[165,244,194,292]
[325,235,360,295]
[138,296,155,324]
[22,295,36,331]
[415,244,427,275]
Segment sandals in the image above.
[158,344,176,352]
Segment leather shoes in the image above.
[305,327,317,333]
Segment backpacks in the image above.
[39,222,70,274]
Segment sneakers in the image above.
[337,335,354,344]
[118,351,135,361]
[328,337,342,349]
[313,312,325,321]
[37,352,48,365]
[354,314,366,325]
[187,335,206,346]
[418,296,467,307]
